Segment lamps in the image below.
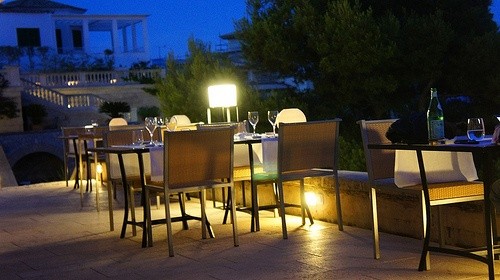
[207,84,240,122]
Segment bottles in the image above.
[427,88,445,141]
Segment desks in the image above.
[85,135,280,248]
[366,137,500,280]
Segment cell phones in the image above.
[454,140,479,144]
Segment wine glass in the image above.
[247,111,259,135]
[267,111,278,136]
[155,116,164,146]
[144,116,158,147]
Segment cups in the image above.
[131,130,144,145]
[467,118,486,141]
[85,124,93,135]
[92,120,99,134]
[167,116,178,131]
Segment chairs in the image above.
[358,117,486,273]
[61,109,344,261]
